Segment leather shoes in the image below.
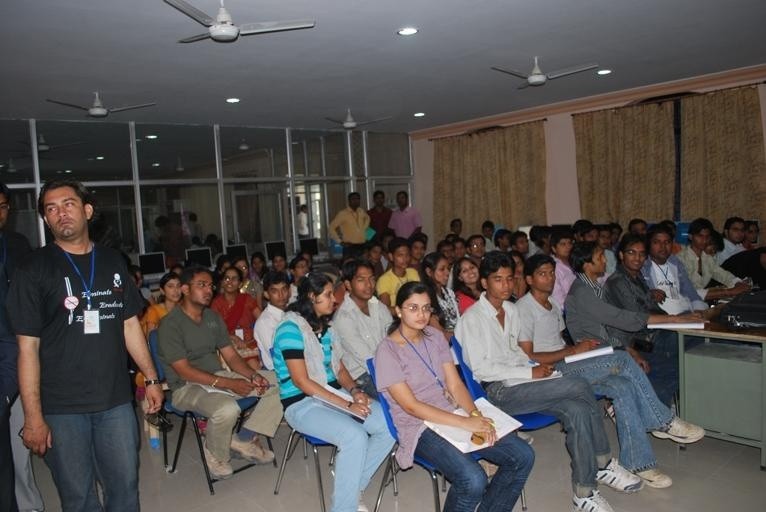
[148,431,160,448]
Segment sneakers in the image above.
[594,457,645,492]
[517,431,534,445]
[572,488,614,512]
[633,467,672,488]
[650,414,705,444]
[202,439,234,480]
[230,432,275,464]
[357,491,369,512]
[194,418,208,434]
[604,401,616,426]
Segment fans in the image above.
[488,56,600,92]
[46,91,157,117]
[163,0,316,45]
[326,106,395,133]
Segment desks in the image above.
[668,305,766,471]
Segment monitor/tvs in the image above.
[138,251,166,280]
[299,237,321,255]
[264,240,289,263]
[225,243,248,261]
[184,247,213,270]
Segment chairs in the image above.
[368,357,498,512]
[151,330,278,494]
[451,335,563,509]
[266,348,373,512]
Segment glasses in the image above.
[402,304,432,313]
[469,243,483,249]
[189,282,217,291]
[242,266,249,270]
[623,250,647,257]
[223,276,239,282]
[142,411,174,432]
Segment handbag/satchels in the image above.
[719,290,766,331]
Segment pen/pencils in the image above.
[251,375,253,384]
[528,360,560,372]
[686,296,695,314]
[471,411,496,428]
[576,338,601,345]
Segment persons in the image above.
[6,177,164,512]
[9,392,45,511]
[296,192,423,261]
[1,181,32,512]
[92,219,766,512]
